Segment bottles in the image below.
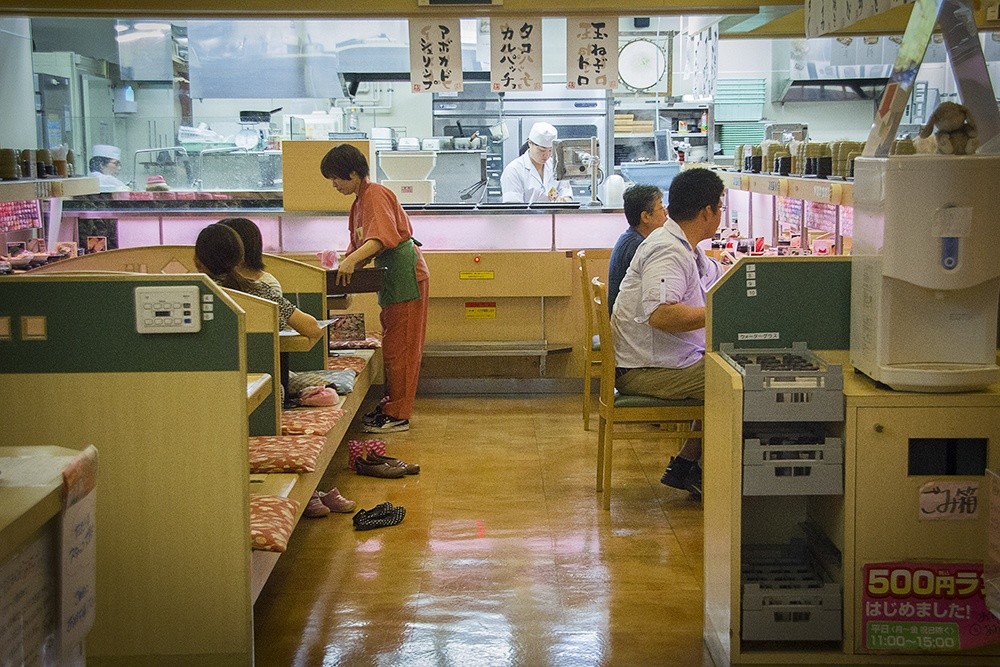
[349,107,360,132]
[700,109,708,134]
[145,175,169,191]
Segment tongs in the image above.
[458,176,490,205]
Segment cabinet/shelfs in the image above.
[703,350,1000,666]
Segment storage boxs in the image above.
[282,141,369,211]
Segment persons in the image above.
[598,167,731,496]
[211,218,283,296]
[318,144,430,433]
[601,184,671,334]
[186,223,321,409]
[86,144,132,195]
[500,122,574,203]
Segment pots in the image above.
[240,107,283,122]
[455,130,481,149]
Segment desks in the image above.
[247,373,273,416]
[280,327,326,408]
[326,294,352,357]
[0,445,99,667]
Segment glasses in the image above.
[104,161,118,166]
[708,205,726,213]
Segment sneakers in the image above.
[660,455,702,496]
[364,416,410,434]
[303,493,330,517]
[362,406,383,423]
[319,489,357,513]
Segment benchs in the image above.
[250,347,380,606]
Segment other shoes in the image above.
[360,505,406,532]
[367,450,419,474]
[355,456,408,477]
[352,501,394,525]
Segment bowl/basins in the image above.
[48,253,68,261]
[2,258,33,265]
[30,254,51,261]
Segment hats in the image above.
[529,122,558,149]
[92,144,121,161]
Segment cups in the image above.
[0,148,75,181]
[734,138,914,179]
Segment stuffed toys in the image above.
[921,103,979,156]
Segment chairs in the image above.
[577,250,601,432]
[591,276,705,510]
